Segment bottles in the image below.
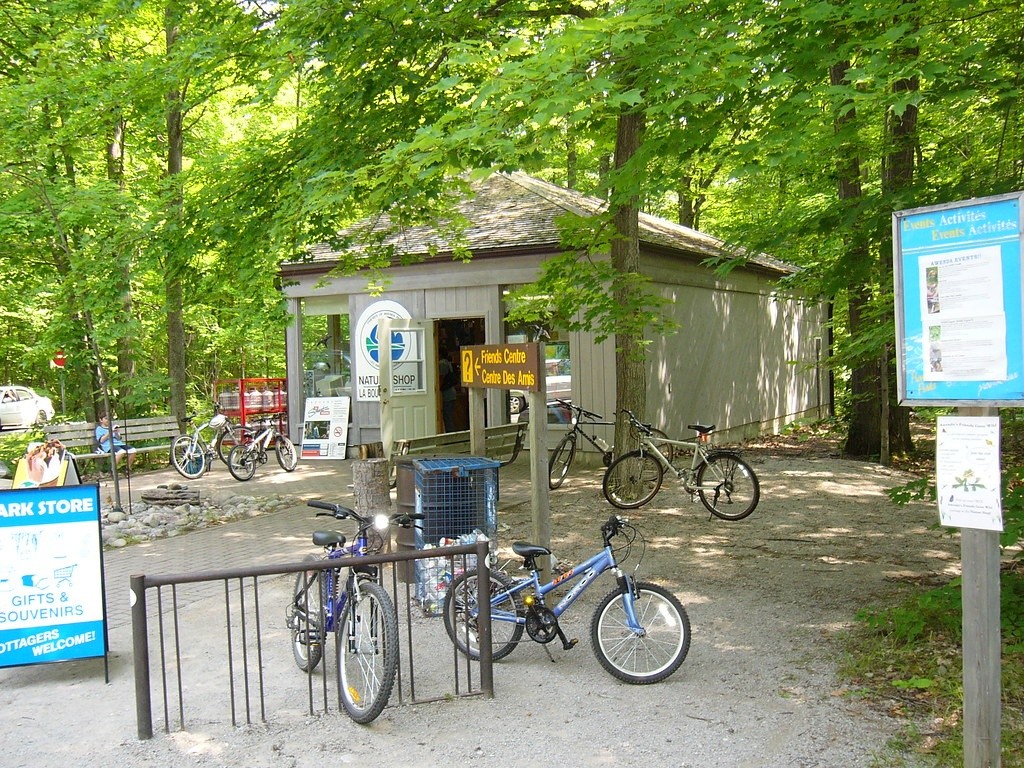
[218,385,288,444]
[592,435,609,451]
[418,531,489,618]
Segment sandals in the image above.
[123,468,129,479]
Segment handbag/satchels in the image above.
[440,372,459,391]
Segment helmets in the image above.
[209,414,227,430]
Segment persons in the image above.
[95,411,137,481]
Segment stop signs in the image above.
[52,352,68,367]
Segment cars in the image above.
[510,360,571,416]
[0,386,55,432]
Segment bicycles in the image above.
[441,515,692,686]
[548,398,674,490]
[170,400,264,481]
[228,412,297,481]
[285,500,427,726]
[603,408,760,523]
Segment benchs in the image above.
[389,422,529,491]
[44,415,190,460]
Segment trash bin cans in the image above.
[411,456,501,617]
